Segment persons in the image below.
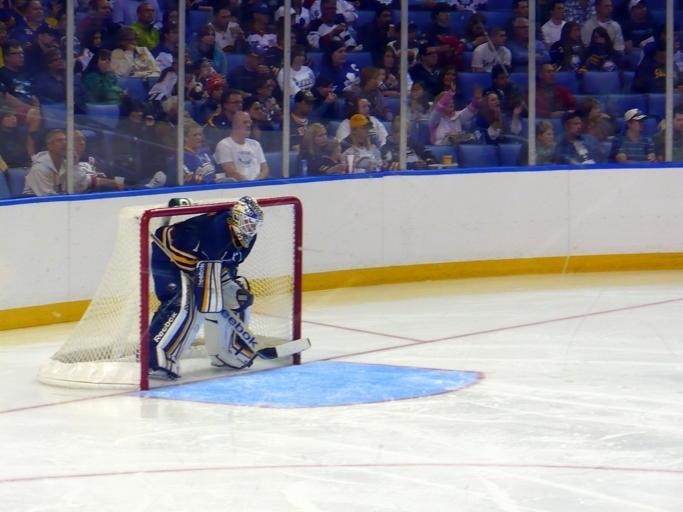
[144,198,262,380]
[0,0,682,199]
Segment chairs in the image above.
[1,0,682,199]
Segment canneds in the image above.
[299,159,309,176]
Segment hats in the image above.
[624,109,647,121]
[351,114,372,128]
[561,107,583,120]
[295,90,318,102]
[252,3,296,19]
[207,76,225,93]
[629,0,641,9]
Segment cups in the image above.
[114,177,124,189]
[442,155,453,164]
[298,160,308,177]
[343,154,354,173]
[367,159,377,173]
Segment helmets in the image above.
[232,196,263,248]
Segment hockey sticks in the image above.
[151,233,310,360]
